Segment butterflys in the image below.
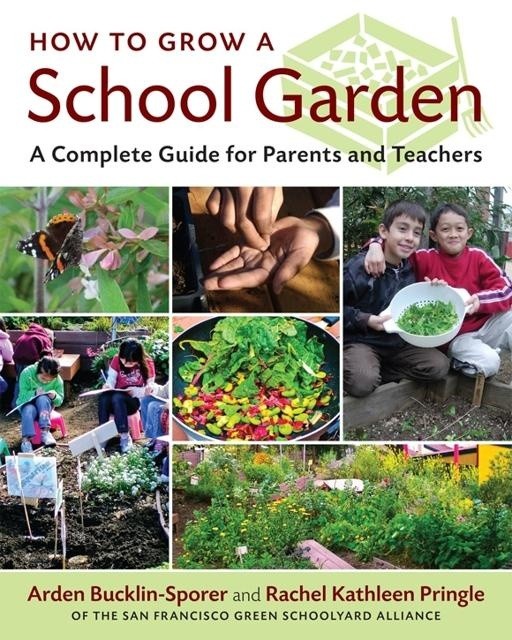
[16,209,83,285]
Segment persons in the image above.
[139,376,171,447]
[343,198,449,397]
[203,187,339,298]
[92,335,155,458]
[360,202,512,380]
[1,319,15,400]
[15,322,54,375]
[13,351,67,454]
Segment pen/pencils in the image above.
[27,377,45,392]
[100,369,111,388]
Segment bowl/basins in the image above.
[382,279,473,348]
[171,317,340,441]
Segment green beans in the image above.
[173,371,330,443]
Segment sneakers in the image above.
[41,431,57,446]
[120,434,133,454]
[21,442,34,453]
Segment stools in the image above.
[0,435,11,467]
[109,410,143,440]
[30,408,67,447]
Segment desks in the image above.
[4,350,81,402]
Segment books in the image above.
[148,392,169,404]
[6,390,51,417]
[78,388,132,398]
